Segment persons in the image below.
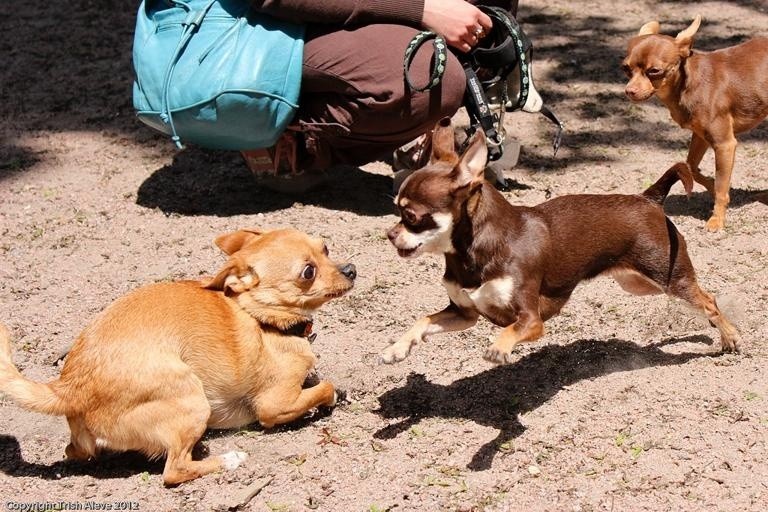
[217,1,518,193]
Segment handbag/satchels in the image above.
[239,130,326,199]
[126,2,305,152]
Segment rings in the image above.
[475,26,484,38]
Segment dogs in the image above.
[621,13,764,233]
[380,115,744,366]
[0,227,357,486]
